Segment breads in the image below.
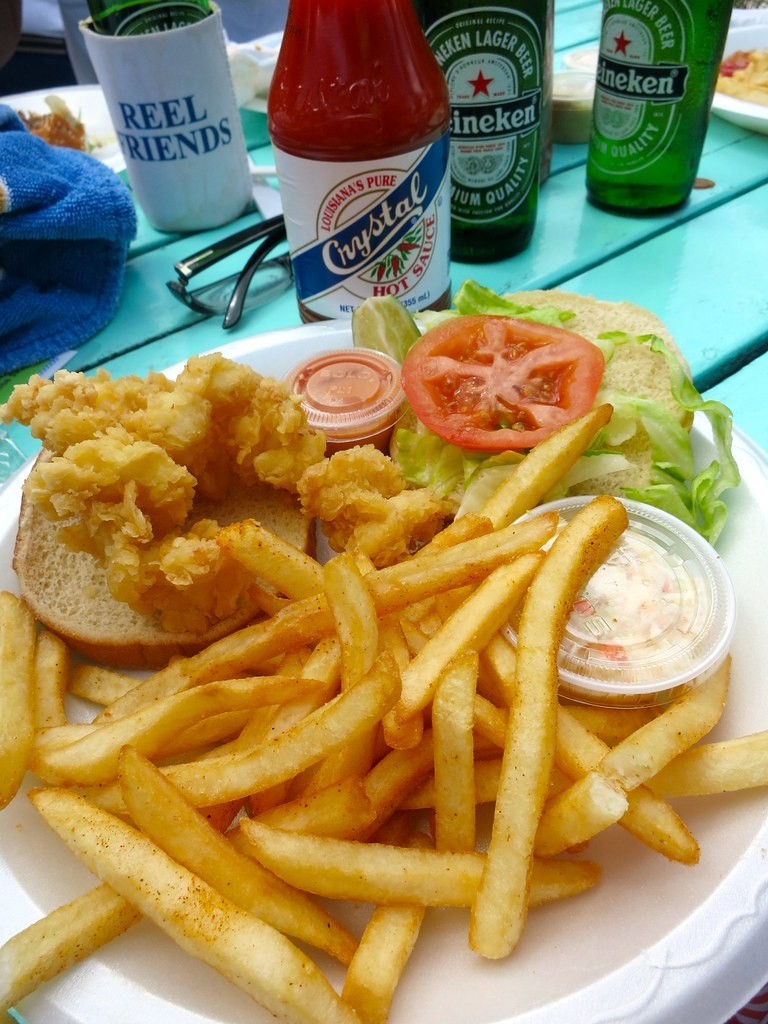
[415,291,691,503]
[15,450,316,670]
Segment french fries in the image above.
[1,404,768,1024]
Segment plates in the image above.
[564,45,600,70]
[712,24,768,135]
[0,313,768,1024]
[0,84,127,176]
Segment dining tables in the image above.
[0,0,767,1023]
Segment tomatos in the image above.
[403,316,604,448]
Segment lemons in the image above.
[353,296,423,364]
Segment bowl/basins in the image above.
[495,496,736,707]
[551,69,597,143]
[281,346,410,457]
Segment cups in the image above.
[78,0,254,234]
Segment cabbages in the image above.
[393,283,741,540]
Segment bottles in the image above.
[416,0,556,267]
[265,0,453,324]
[82,0,211,39]
[586,0,734,218]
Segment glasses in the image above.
[165,215,295,332]
